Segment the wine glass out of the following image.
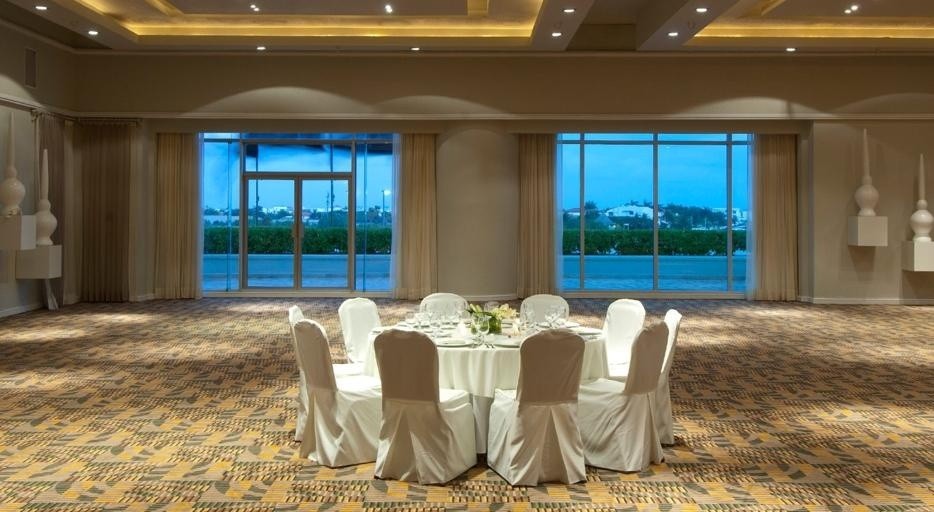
[372,300,601,348]
